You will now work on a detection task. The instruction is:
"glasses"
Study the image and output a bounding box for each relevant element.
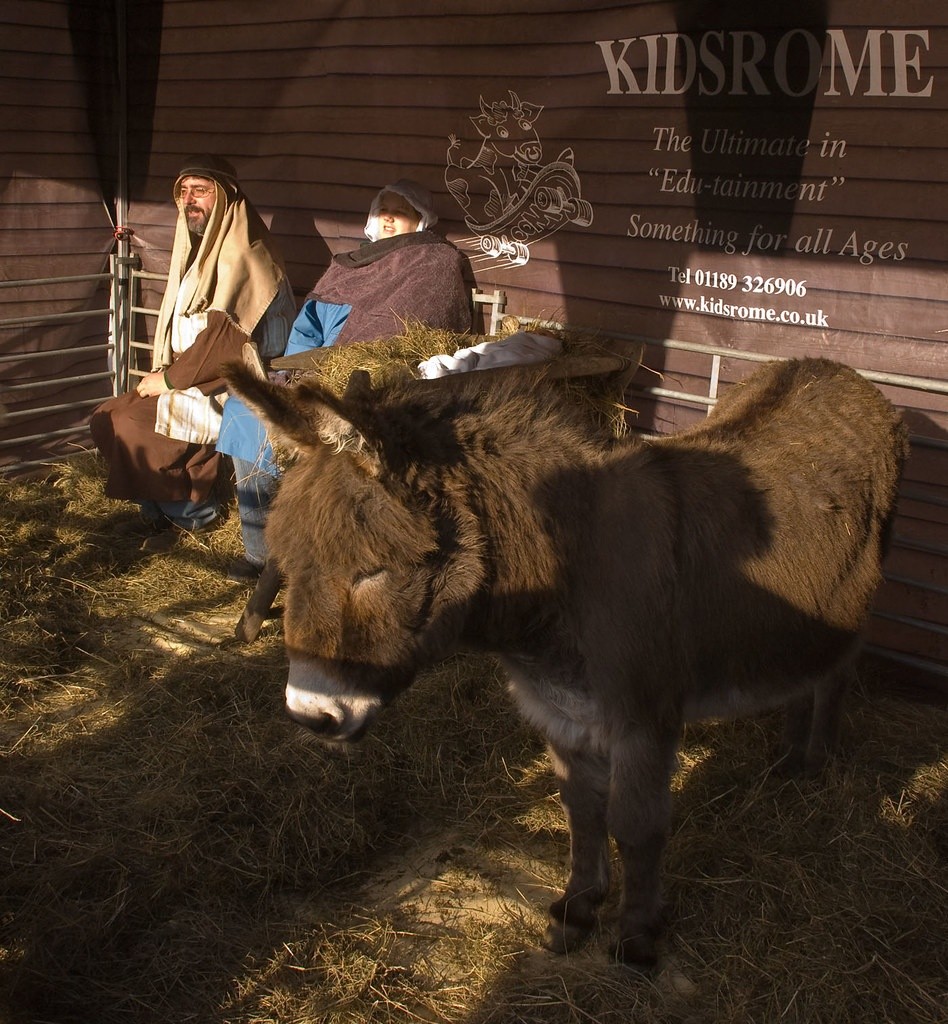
[177,187,215,198]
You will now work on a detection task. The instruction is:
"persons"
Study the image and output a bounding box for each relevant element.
[90,153,297,540]
[216,177,472,587]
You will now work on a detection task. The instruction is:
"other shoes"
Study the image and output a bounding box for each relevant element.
[143,513,219,553]
[226,558,261,578]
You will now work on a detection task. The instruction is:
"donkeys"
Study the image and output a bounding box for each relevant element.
[221,355,911,975]
